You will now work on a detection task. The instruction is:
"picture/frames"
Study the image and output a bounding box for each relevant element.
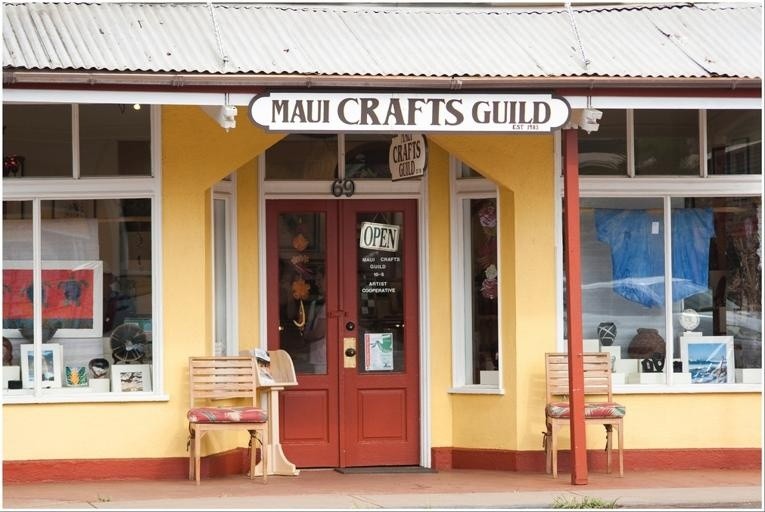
[680,335,736,384]
[2,259,152,392]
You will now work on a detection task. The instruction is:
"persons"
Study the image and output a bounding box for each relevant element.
[3,335,13,366]
[301,266,328,374]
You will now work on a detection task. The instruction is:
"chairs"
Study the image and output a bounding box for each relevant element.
[188,356,269,486]
[543,351,626,480]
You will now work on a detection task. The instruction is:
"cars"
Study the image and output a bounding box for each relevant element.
[563,277,762,370]
